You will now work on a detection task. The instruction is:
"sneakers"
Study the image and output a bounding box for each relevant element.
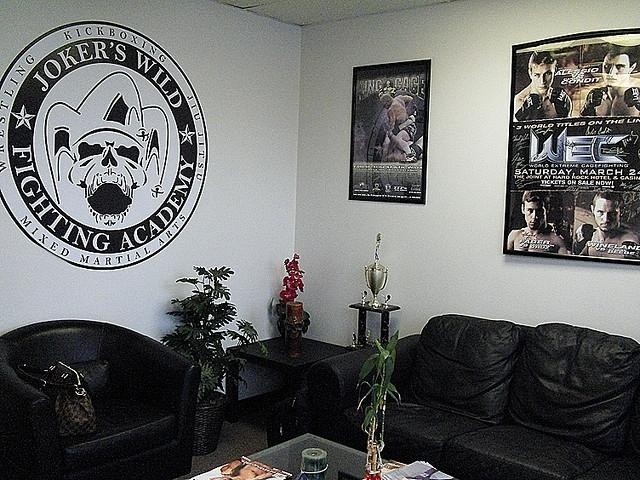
[399,156,417,163]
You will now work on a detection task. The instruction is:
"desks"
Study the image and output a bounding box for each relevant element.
[227,338,350,429]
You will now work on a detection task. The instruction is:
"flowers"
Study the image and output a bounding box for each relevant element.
[278,252,303,303]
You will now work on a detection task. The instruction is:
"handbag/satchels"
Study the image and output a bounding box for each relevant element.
[67,361,96,394]
[55,387,96,436]
[14,358,92,406]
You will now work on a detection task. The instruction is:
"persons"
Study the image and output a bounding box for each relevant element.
[514,51,573,121]
[212,460,274,480]
[379,93,417,164]
[580,50,640,117]
[575,192,639,258]
[508,190,571,254]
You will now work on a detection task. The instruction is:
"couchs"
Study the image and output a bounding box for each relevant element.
[306,314,639,480]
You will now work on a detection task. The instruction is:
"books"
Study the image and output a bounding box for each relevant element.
[381,458,454,480]
[189,455,293,480]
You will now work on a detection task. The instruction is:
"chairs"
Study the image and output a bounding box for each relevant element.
[1,318,200,479]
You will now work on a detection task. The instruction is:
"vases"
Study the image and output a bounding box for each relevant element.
[275,300,295,318]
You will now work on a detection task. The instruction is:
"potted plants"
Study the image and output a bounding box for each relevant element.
[159,264,272,457]
[355,331,399,480]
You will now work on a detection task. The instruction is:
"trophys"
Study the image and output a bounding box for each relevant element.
[363,232,389,309]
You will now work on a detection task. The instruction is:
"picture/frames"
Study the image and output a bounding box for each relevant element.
[503,27,640,266]
[349,58,431,205]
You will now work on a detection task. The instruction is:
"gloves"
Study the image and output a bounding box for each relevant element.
[622,241,640,259]
[550,87,571,117]
[574,223,596,254]
[516,94,542,121]
[553,220,572,251]
[579,88,602,116]
[624,87,640,111]
